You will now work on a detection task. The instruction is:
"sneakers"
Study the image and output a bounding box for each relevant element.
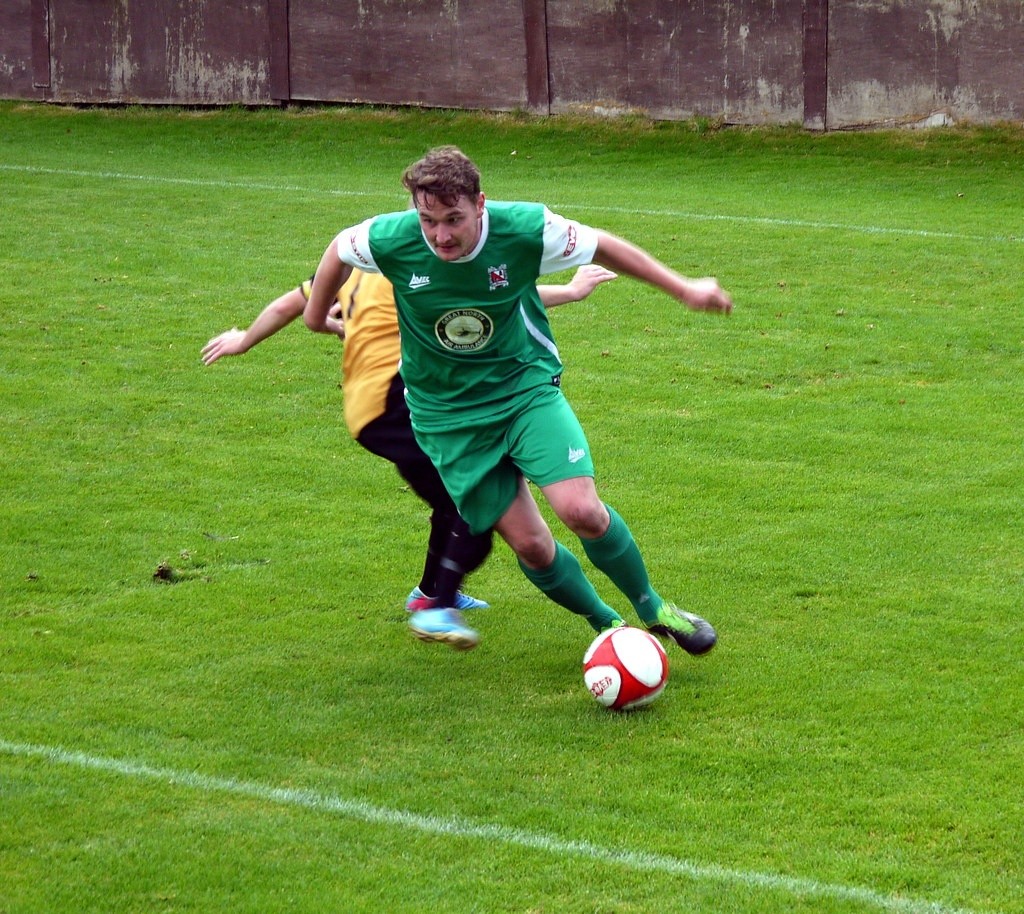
[404,585,491,615]
[640,598,719,657]
[597,620,629,636]
[406,606,481,649]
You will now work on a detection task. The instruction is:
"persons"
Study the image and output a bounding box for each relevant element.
[201,264,618,650]
[304,145,733,658]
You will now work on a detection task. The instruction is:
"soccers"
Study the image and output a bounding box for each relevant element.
[582,626,669,709]
